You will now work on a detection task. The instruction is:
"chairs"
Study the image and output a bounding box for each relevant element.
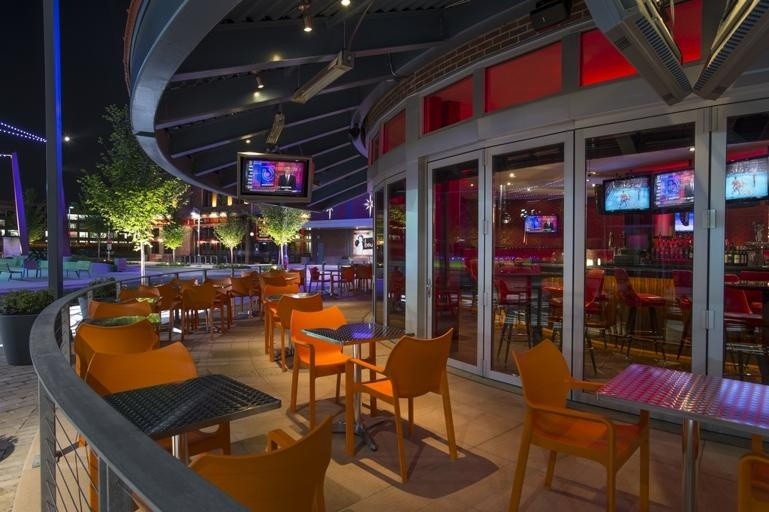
[508,337,649,512]
[738,435,769,512]
[263,284,301,355]
[290,307,376,429]
[85,287,161,326]
[131,414,333,512]
[74,319,161,448]
[155,263,373,341]
[83,343,231,512]
[345,327,457,482]
[389,242,769,375]
[274,295,323,370]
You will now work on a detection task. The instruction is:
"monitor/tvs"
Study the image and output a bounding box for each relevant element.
[653,167,695,215]
[725,154,769,204]
[237,151,315,204]
[602,174,653,215]
[523,214,559,234]
[673,211,694,234]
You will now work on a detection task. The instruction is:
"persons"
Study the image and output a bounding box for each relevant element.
[544,216,553,232]
[277,165,296,190]
[390,266,403,304]
[619,190,629,208]
[731,176,742,195]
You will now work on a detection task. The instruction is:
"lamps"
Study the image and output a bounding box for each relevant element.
[292,21,354,106]
[298,4,312,32]
[256,77,264,89]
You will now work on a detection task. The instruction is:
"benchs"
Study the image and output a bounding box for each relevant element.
[6,259,91,281]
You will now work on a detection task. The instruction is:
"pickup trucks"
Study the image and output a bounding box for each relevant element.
[493,265,544,305]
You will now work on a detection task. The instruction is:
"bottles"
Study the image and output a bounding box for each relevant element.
[651,236,769,271]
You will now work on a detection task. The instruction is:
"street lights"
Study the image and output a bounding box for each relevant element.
[68,207,73,252]
[190,206,202,267]
[283,207,289,272]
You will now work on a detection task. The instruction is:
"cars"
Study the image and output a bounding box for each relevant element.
[100,249,114,256]
[447,273,473,291]
[401,278,460,318]
[663,270,693,321]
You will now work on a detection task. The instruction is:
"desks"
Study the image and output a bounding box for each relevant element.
[300,324,415,451]
[102,373,282,466]
[598,361,769,512]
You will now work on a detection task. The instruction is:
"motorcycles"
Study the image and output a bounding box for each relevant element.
[388,283,405,314]
